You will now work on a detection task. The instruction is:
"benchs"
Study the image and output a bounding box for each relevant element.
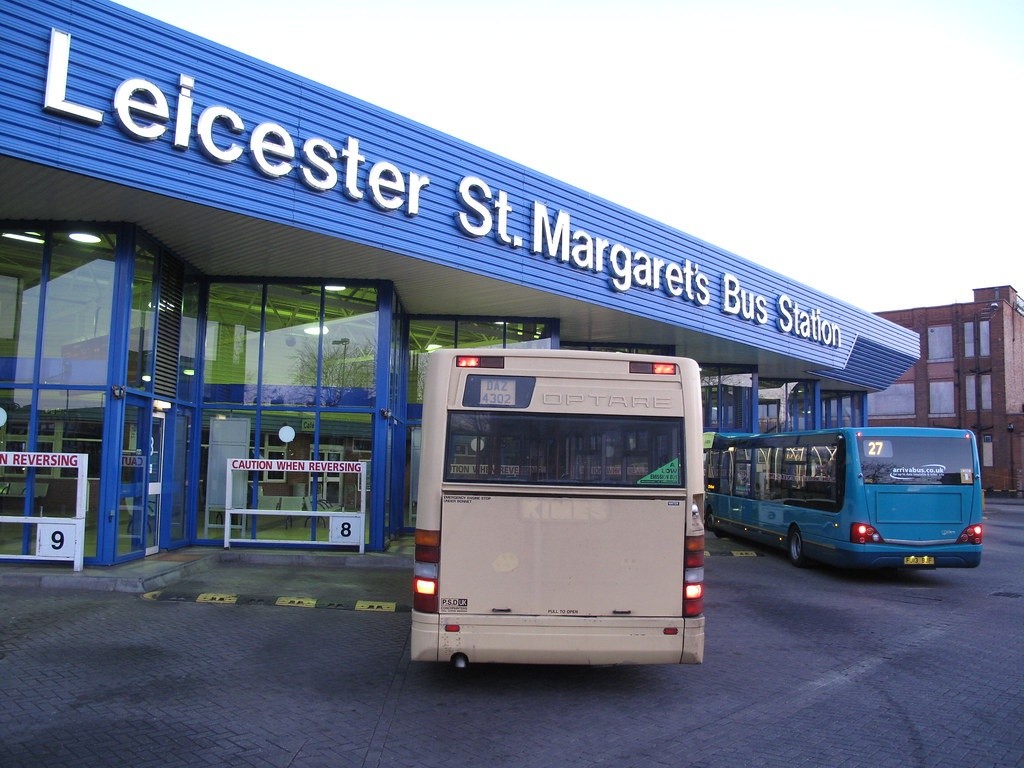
[0,482,49,517]
[257,496,304,530]
[304,495,341,528]
[124,497,156,534]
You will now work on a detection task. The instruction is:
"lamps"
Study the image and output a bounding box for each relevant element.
[110,383,127,400]
[380,405,393,419]
[279,416,296,443]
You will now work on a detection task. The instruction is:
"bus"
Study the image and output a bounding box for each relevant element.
[704,431,982,574]
[408,348,706,668]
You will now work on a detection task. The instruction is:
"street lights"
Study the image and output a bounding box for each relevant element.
[332,337,351,388]
